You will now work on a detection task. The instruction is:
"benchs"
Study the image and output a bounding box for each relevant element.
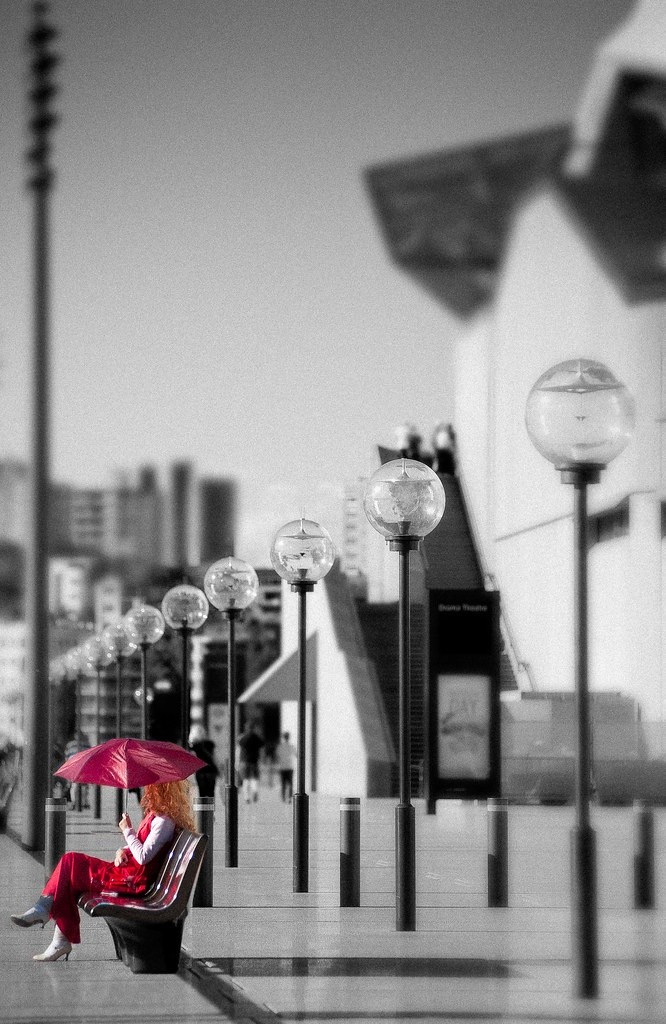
[75,832,211,973]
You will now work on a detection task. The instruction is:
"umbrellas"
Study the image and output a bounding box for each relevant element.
[52,737,211,817]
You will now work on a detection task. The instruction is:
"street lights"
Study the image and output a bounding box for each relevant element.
[203,551,260,868]
[361,458,446,933]
[159,582,211,753]
[97,621,139,829]
[62,646,89,812]
[49,658,65,800]
[121,602,166,739]
[266,518,340,895]
[82,634,117,819]
[524,356,640,998]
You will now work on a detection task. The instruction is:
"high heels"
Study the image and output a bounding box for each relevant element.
[32,924,73,961]
[10,895,54,930]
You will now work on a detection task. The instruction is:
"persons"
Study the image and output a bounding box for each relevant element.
[275,733,297,803]
[9,773,196,961]
[187,733,219,799]
[238,721,264,804]
